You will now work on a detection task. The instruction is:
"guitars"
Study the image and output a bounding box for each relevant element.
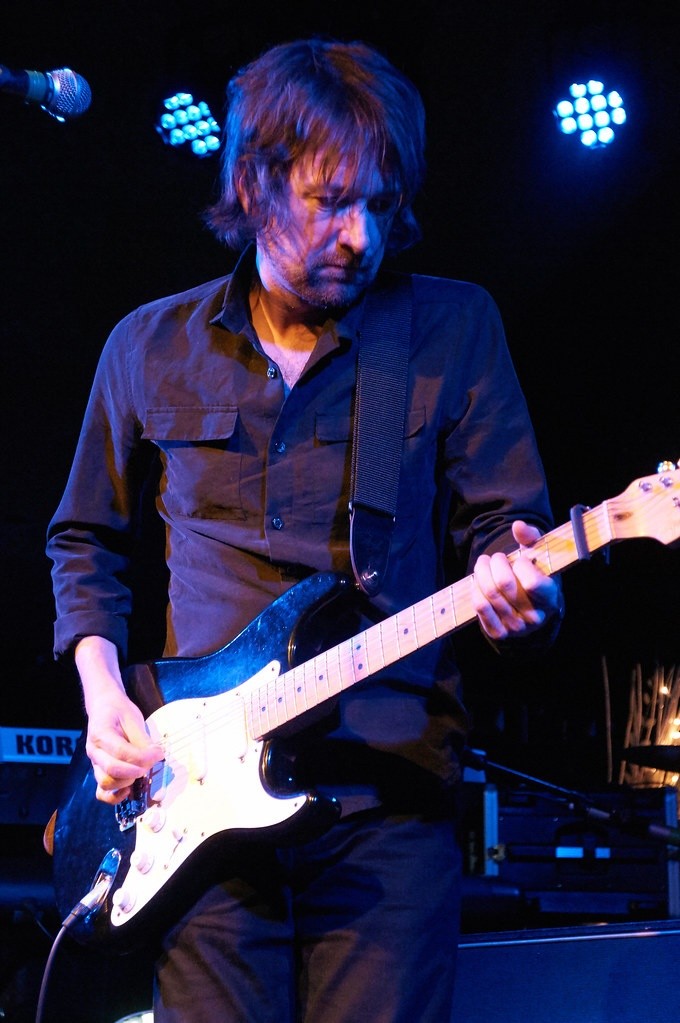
[50,454,679,956]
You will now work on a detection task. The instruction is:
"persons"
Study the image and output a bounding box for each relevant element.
[44,36,567,1023]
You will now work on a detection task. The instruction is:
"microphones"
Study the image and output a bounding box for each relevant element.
[0,65,92,121]
